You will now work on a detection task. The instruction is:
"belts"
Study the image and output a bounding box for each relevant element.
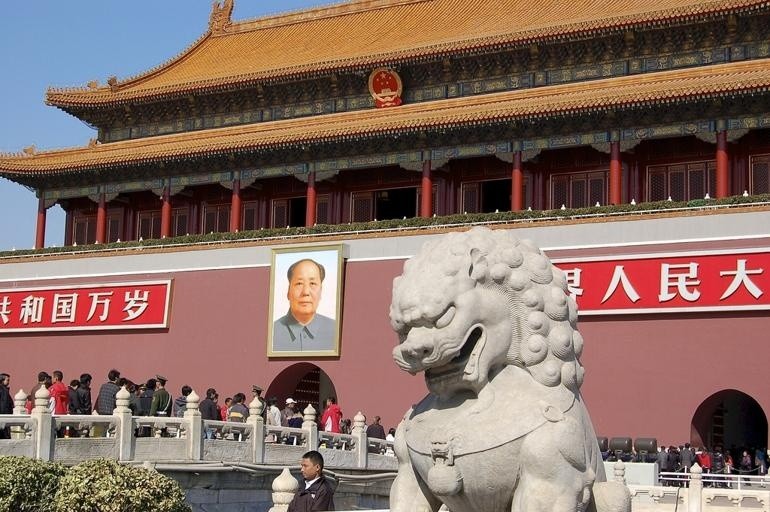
[156,411,168,414]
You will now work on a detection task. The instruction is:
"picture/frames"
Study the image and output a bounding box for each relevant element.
[265,243,344,361]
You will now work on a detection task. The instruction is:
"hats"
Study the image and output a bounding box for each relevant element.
[137,383,146,390]
[285,398,297,405]
[252,385,264,392]
[156,375,168,382]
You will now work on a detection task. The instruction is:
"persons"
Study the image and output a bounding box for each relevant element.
[601,443,770,489]
[287,451,335,512]
[273,260,336,351]
